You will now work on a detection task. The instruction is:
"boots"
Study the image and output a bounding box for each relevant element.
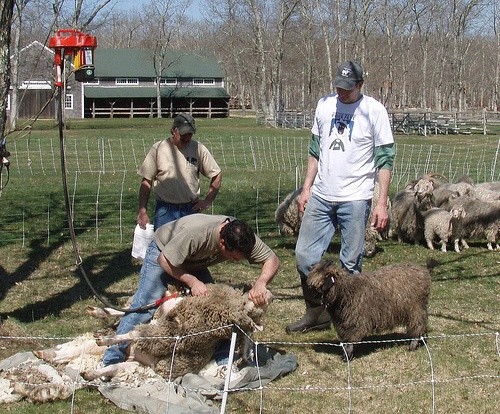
[286,277,331,335]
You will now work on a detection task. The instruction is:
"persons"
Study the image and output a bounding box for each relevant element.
[97,214,280,382]
[137,113,222,233]
[286,61,396,336]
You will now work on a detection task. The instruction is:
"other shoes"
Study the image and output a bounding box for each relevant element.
[218,363,239,379]
[97,362,112,382]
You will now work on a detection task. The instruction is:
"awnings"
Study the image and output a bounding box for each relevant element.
[84,87,231,99]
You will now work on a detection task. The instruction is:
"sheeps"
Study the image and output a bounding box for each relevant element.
[422,204,470,253]
[81,281,274,382]
[306,259,432,362]
[274,171,499,257]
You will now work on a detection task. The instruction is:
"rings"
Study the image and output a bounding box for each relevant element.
[200,208,201,210]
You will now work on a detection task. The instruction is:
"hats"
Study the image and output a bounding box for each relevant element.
[173,114,196,136]
[334,60,362,91]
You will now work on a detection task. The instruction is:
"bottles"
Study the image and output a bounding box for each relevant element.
[131,223,154,260]
